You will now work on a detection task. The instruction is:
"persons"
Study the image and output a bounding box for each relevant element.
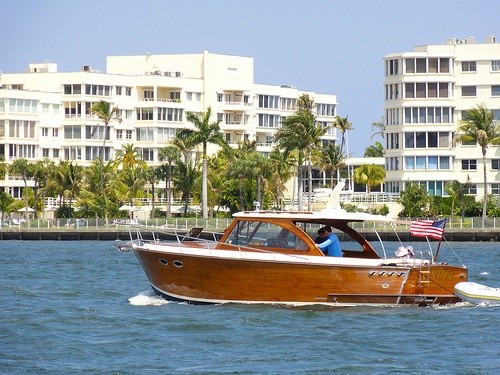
[315,228,328,256]
[312,225,344,257]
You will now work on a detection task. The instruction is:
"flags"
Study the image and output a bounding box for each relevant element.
[410,218,449,240]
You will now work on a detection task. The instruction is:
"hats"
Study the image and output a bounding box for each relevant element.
[394,246,408,257]
[406,245,415,256]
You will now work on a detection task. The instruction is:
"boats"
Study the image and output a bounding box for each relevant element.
[453,281,500,306]
[130,209,470,308]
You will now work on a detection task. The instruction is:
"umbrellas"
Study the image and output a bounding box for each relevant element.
[16,206,35,212]
